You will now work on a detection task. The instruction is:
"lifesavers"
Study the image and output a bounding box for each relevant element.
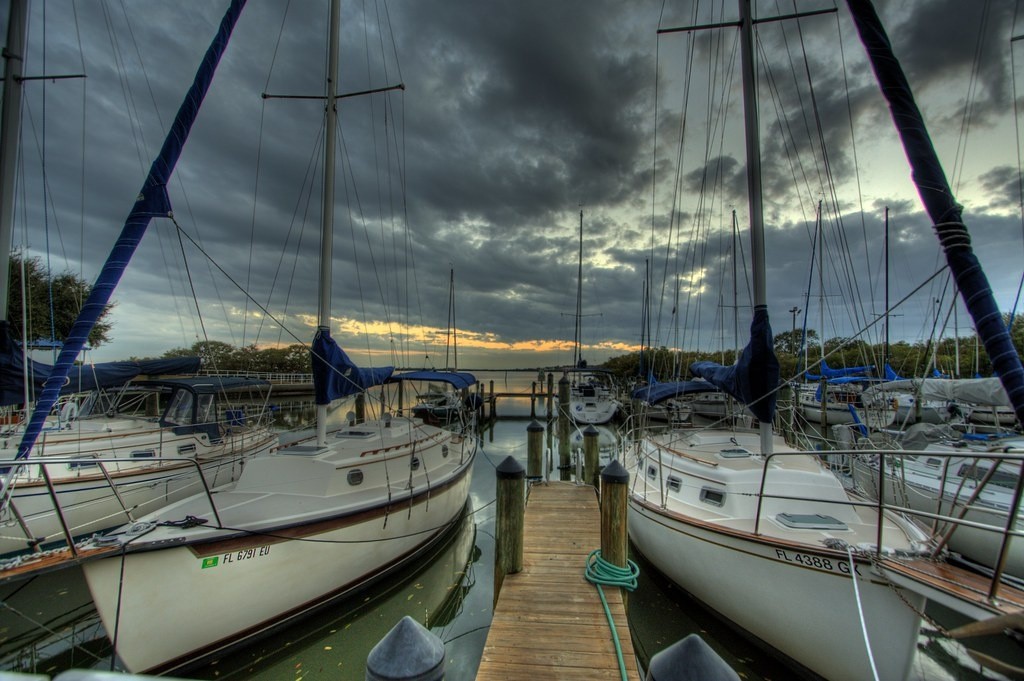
[59,401,77,422]
[892,398,898,408]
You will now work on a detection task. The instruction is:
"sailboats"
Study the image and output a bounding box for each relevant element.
[0,1,1024,681]
